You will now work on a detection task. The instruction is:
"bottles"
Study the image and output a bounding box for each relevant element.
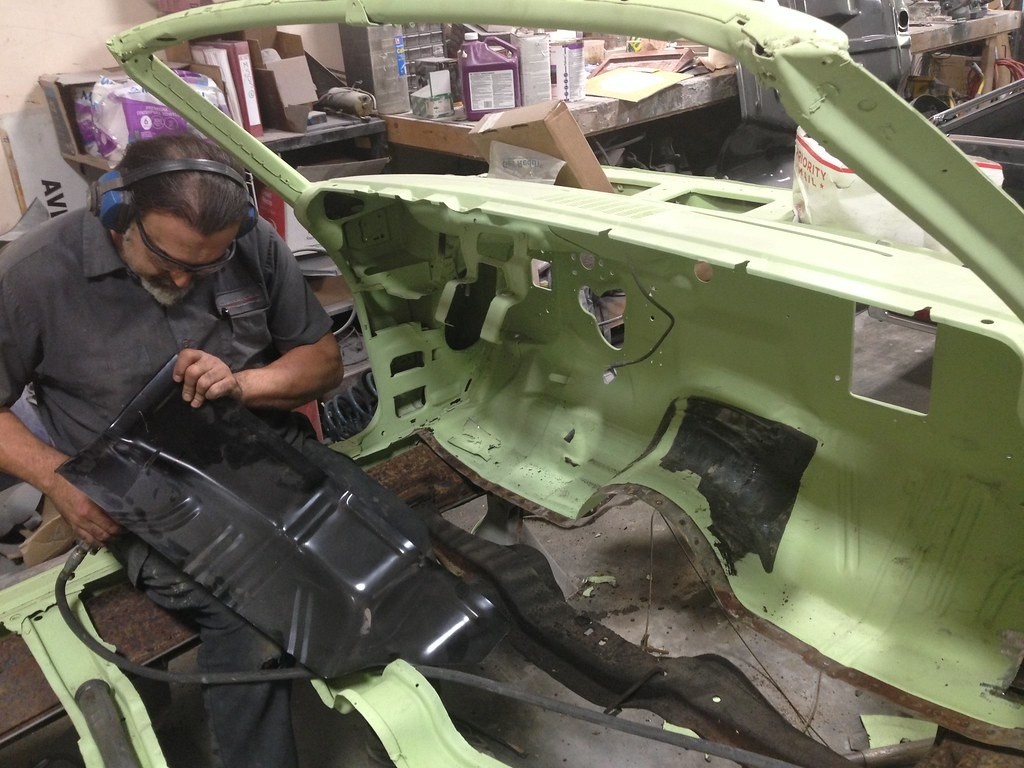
[457,32,522,122]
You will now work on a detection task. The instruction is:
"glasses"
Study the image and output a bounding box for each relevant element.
[132,214,238,278]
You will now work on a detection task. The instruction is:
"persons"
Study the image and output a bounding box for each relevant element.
[0,135,343,767]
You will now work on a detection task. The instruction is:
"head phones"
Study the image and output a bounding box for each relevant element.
[82,158,259,241]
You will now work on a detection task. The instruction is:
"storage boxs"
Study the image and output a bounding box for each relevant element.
[466,99,616,193]
[255,157,390,258]
[38,1,324,170]
